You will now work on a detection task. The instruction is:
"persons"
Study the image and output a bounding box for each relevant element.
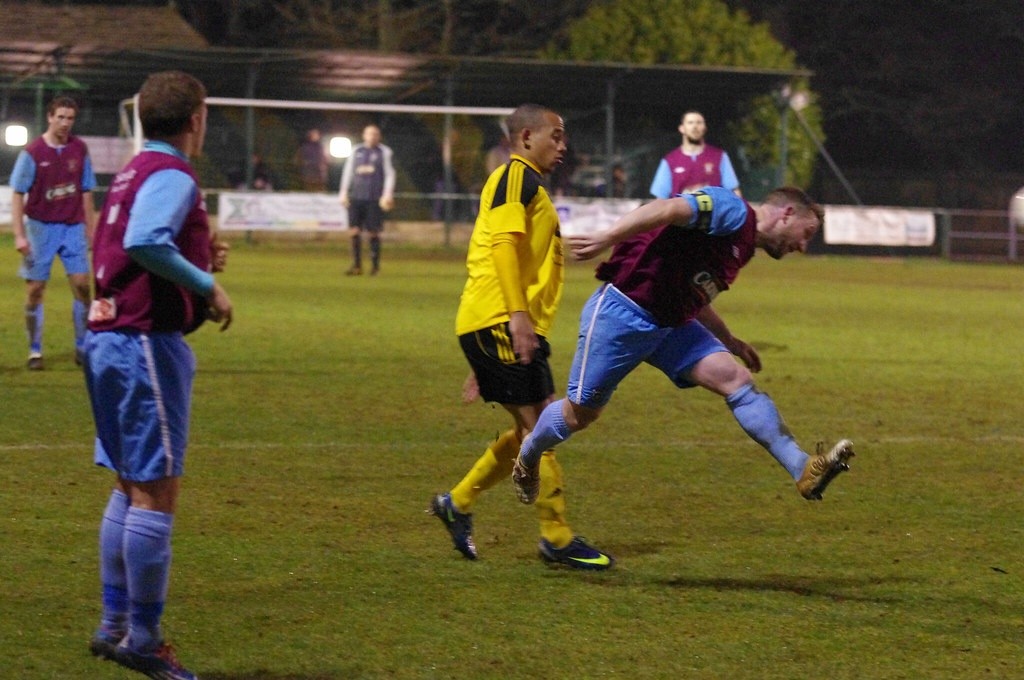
[431,105,615,572]
[10,96,98,371]
[339,124,395,277]
[650,110,742,200]
[513,187,857,505]
[238,128,643,220]
[82,71,233,680]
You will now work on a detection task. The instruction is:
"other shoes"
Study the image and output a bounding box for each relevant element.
[371,266,379,276]
[347,267,363,275]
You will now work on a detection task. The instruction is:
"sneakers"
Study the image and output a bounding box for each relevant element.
[27,353,42,370]
[113,634,198,680]
[536,535,616,570]
[75,350,84,364]
[796,439,856,501]
[512,432,540,505]
[89,619,128,661]
[425,491,480,560]
